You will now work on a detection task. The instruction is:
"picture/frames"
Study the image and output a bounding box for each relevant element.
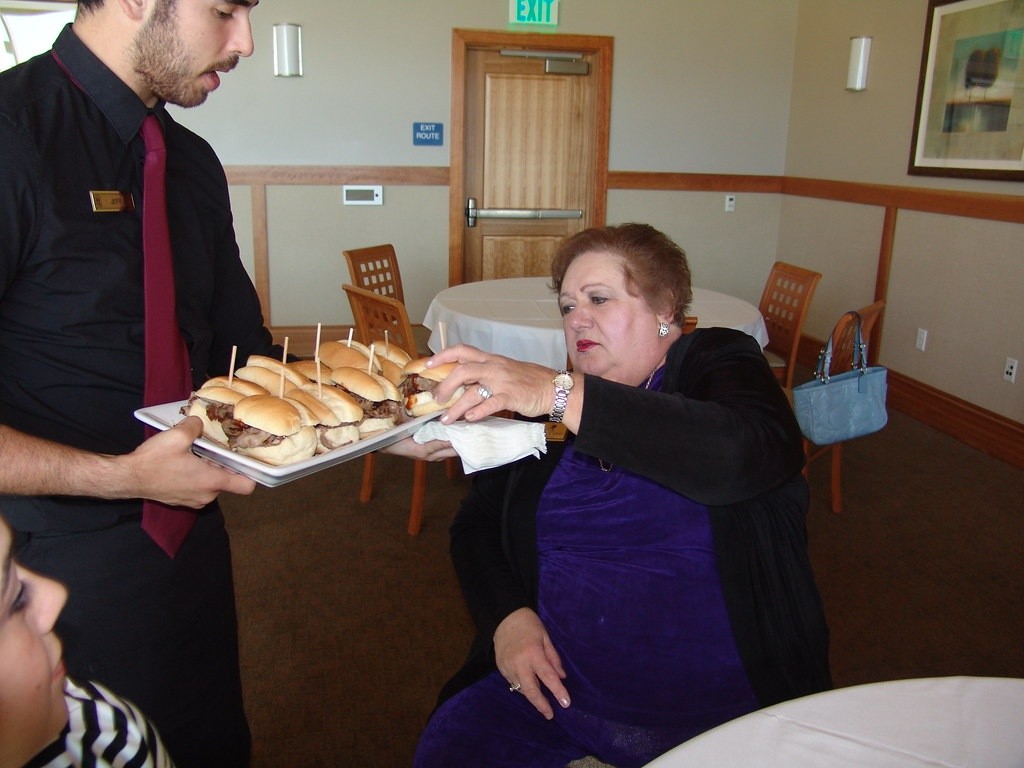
[906,0,1024,183]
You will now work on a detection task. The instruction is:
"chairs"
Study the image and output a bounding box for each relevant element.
[761,261,822,389]
[777,301,885,514]
[341,284,515,536]
[566,317,698,371]
[341,244,428,360]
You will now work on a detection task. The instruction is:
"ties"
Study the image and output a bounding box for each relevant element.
[53,54,201,559]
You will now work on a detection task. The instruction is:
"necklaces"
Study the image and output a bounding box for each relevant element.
[599,368,657,472]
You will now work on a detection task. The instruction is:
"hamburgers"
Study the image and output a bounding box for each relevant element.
[179,340,474,467]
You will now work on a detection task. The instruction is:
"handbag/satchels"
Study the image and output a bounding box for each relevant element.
[790,310,887,445]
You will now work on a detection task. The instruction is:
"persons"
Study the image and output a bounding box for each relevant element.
[0,0,461,768]
[410,223,831,768]
[0,516,176,768]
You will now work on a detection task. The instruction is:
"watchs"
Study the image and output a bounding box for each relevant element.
[549,370,574,423]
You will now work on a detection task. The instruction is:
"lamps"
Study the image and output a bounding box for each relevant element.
[271,23,304,77]
[844,35,872,92]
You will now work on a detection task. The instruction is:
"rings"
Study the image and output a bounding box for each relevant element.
[479,385,491,399]
[509,684,522,692]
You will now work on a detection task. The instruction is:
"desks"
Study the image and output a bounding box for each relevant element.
[423,277,769,374]
[640,675,1024,768]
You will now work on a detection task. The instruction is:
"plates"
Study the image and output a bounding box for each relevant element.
[134,400,450,488]
